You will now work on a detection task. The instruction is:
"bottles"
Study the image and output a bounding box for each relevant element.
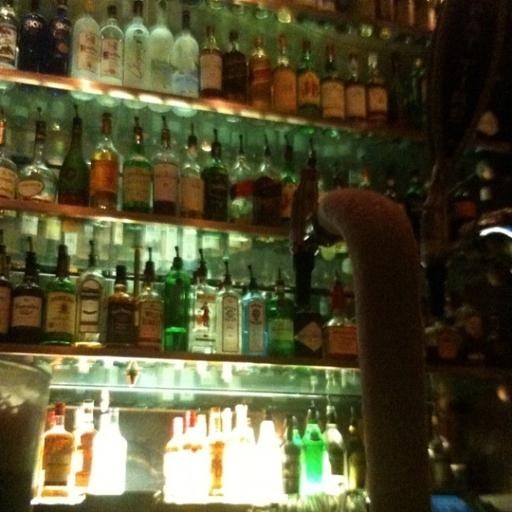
[366,56,390,132]
[187,269,220,354]
[202,141,230,220]
[73,1,100,82]
[468,333,509,368]
[42,244,76,347]
[255,145,281,226]
[0,246,12,342]
[323,423,347,509]
[162,257,189,351]
[359,172,372,191]
[407,176,430,240]
[478,187,495,218]
[216,273,242,355]
[249,35,271,111]
[135,261,164,351]
[60,116,89,207]
[273,32,296,115]
[75,255,105,347]
[0,0,18,71]
[43,413,54,434]
[455,301,483,342]
[0,121,17,200]
[346,52,367,127]
[268,286,294,358]
[162,403,300,508]
[452,182,476,229]
[223,30,249,103]
[241,279,270,357]
[124,1,149,87]
[106,265,136,347]
[46,0,71,76]
[296,38,322,120]
[280,145,302,225]
[72,405,97,496]
[38,403,76,498]
[123,126,152,213]
[425,321,462,363]
[323,287,360,363]
[385,177,399,204]
[90,112,120,212]
[20,121,57,203]
[230,154,253,225]
[181,135,204,219]
[100,4,122,85]
[151,1,173,95]
[306,150,316,168]
[293,313,326,358]
[173,10,199,100]
[22,0,44,73]
[151,128,180,216]
[89,407,128,496]
[10,252,45,344]
[200,25,222,97]
[426,411,470,494]
[322,45,346,124]
[302,419,325,496]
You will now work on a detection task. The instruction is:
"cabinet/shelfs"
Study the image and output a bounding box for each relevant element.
[1,0,512,512]
